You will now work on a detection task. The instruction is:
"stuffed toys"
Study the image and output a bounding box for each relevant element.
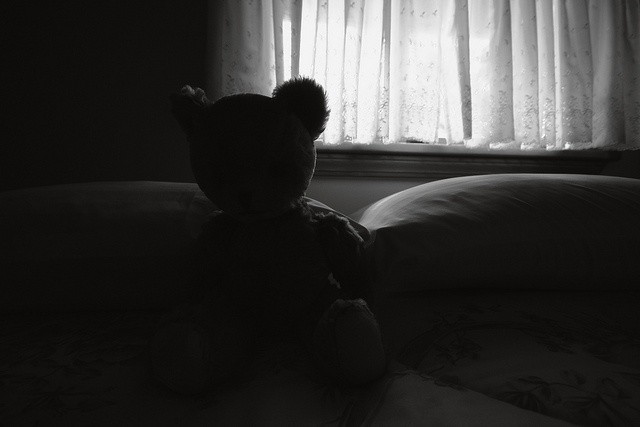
[148,79,388,399]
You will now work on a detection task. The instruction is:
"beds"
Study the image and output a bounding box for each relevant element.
[0,179,640,426]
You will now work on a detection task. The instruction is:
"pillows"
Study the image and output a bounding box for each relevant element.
[344,171,640,298]
[0,181,373,310]
